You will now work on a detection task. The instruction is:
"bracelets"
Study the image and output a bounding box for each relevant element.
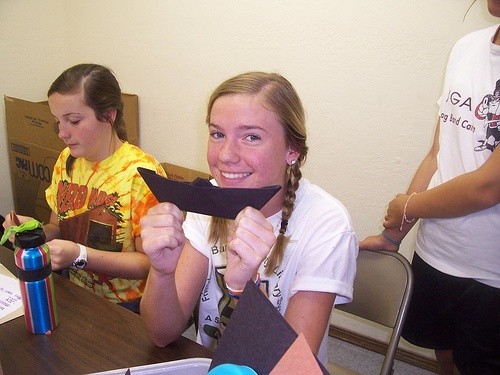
[400,192,417,231]
[226,272,261,299]
[381,231,401,245]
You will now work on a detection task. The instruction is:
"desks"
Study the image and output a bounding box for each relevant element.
[0,245,216,375]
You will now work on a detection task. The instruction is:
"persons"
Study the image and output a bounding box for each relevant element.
[356,0,500,375]
[2,63,170,316]
[140,71,359,366]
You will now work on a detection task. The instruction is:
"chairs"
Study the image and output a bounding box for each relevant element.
[327,249,414,375]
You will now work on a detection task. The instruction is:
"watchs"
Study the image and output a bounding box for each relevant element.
[72,243,88,270]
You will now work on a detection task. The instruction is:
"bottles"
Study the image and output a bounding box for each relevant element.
[13,227,59,335]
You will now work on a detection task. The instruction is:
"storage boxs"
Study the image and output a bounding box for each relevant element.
[3,93,141,225]
